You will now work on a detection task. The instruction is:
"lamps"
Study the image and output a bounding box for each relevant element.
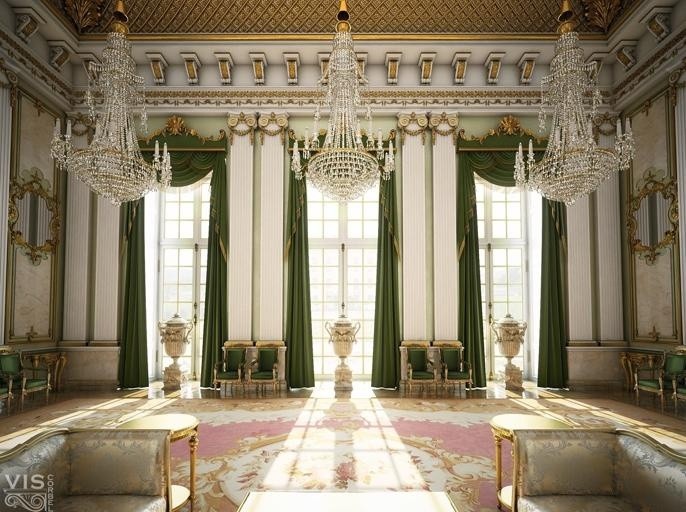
[48,0,174,208]
[290,0,396,206]
[512,0,636,208]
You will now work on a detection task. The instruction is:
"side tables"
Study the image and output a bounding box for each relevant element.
[24,351,69,393]
[488,413,571,512]
[619,351,662,393]
[116,414,200,512]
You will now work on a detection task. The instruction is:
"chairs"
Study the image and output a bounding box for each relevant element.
[0,344,50,413]
[672,372,686,414]
[247,348,279,396]
[213,348,247,393]
[0,374,18,417]
[438,347,473,395]
[632,345,686,413]
[406,348,435,393]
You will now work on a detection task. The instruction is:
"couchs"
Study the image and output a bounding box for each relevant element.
[0,428,172,512]
[511,427,686,512]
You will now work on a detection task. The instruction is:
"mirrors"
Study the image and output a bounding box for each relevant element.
[625,166,678,265]
[8,167,63,266]
